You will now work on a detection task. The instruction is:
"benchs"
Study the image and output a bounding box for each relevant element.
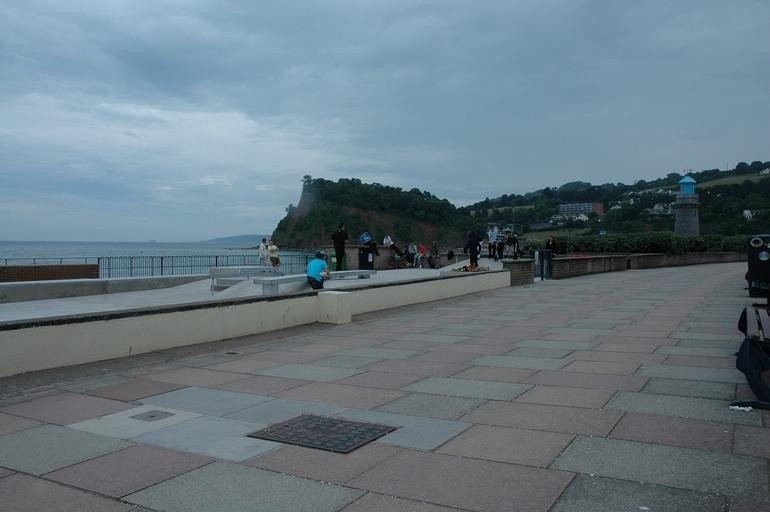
[253,270,376,297]
[389,257,440,268]
[211,267,283,297]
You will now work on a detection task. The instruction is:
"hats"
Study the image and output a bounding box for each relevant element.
[316,251,325,258]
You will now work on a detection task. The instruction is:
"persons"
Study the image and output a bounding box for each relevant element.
[545,234,556,256]
[258,238,271,273]
[268,240,281,268]
[359,229,381,256]
[329,221,349,271]
[406,240,439,268]
[304,250,331,290]
[463,225,526,268]
[383,233,404,257]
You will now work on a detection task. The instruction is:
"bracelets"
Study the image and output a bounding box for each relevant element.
[326,272,329,274]
